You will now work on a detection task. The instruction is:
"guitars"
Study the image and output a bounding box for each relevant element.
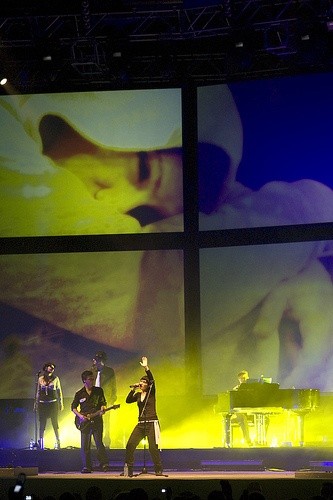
[75,404,120,430]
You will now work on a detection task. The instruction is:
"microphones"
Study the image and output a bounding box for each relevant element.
[130,384,142,387]
[35,370,41,376]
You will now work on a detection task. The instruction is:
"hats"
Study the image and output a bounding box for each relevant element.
[43,363,55,371]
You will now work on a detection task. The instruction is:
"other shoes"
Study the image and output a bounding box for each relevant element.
[155,470,162,475]
[121,469,133,476]
[247,440,253,446]
[101,464,109,471]
[54,442,60,451]
[81,466,90,473]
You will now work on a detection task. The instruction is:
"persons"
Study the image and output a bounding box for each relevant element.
[119,355,163,476]
[233,370,269,446]
[71,370,109,474]
[91,351,117,447]
[0,85,333,400]
[32,362,64,450]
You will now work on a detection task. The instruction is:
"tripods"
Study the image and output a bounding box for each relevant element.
[130,388,168,477]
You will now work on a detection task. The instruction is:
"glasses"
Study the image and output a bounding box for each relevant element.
[140,381,147,383]
[87,378,95,381]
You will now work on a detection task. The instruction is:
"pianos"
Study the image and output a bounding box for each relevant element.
[213,377,321,448]
